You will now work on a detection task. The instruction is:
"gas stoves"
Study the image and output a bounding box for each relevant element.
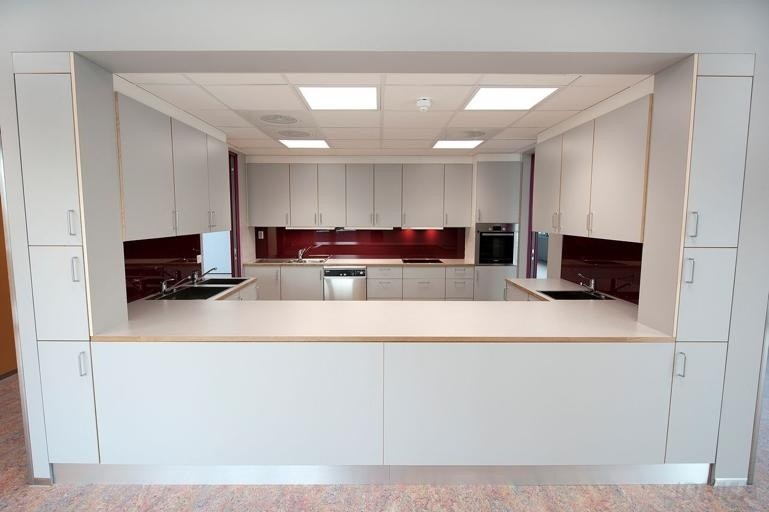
[401,256,445,265]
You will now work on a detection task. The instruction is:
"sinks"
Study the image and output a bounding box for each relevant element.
[200,278,246,284]
[540,290,615,300]
[255,259,287,263]
[286,258,324,264]
[160,287,230,300]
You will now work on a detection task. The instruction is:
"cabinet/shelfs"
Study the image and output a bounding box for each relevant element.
[678,251,737,342]
[683,76,753,248]
[28,246,90,341]
[14,72,81,246]
[36,340,100,464]
[664,340,728,466]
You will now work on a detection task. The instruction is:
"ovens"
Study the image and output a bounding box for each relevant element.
[476,223,519,266]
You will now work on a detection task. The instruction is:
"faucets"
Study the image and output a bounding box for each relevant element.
[578,272,599,292]
[298,247,310,260]
[159,275,191,294]
[192,267,217,283]
[610,272,636,293]
